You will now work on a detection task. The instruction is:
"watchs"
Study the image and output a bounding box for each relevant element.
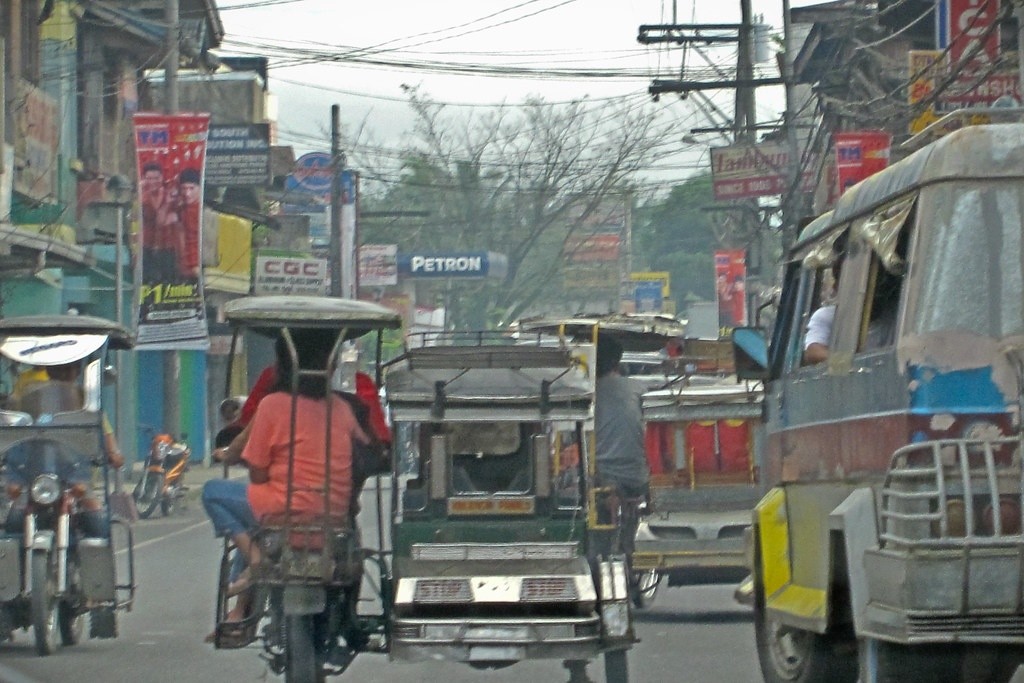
[222,447,229,457]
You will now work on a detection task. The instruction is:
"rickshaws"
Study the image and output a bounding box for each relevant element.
[211,295,642,683]
[582,337,764,608]
[0,309,139,657]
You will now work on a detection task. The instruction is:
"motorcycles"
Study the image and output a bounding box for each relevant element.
[730,117,1023,683]
[131,429,192,518]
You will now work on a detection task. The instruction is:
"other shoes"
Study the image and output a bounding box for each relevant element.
[205,612,251,642]
[226,556,276,598]
[339,617,372,649]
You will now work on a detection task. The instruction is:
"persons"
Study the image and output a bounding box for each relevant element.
[203,327,391,645]
[799,246,901,363]
[558,332,647,575]
[133,229,143,231]
[140,162,199,306]
[11,358,125,482]
[719,268,746,327]
